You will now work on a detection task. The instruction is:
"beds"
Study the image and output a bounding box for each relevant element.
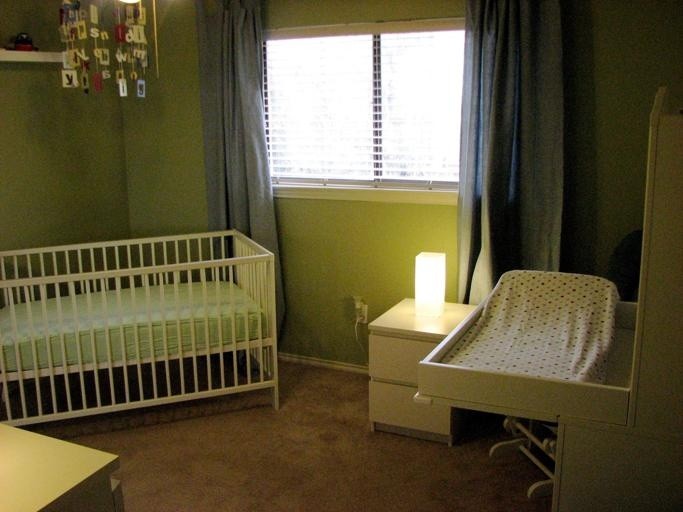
[0,229,280,426]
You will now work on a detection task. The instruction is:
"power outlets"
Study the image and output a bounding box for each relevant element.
[355,305,368,325]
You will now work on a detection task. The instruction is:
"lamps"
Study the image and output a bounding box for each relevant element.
[415,252,446,317]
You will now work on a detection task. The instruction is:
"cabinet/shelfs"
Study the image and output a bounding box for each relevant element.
[413,82,683,512]
[367,298,481,447]
[0,422,125,512]
